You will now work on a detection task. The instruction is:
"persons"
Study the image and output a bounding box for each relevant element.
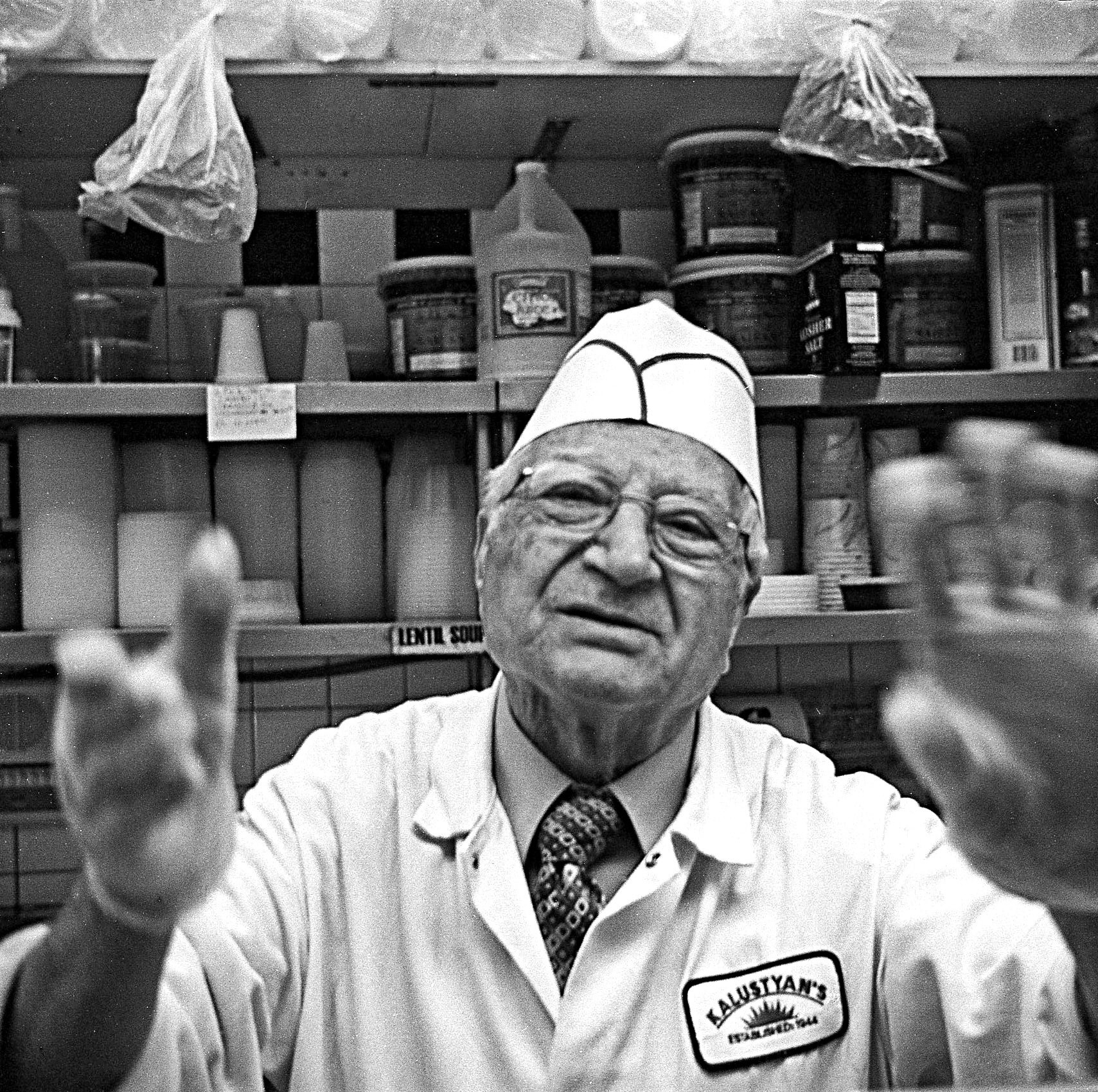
[0,297,1097,1092]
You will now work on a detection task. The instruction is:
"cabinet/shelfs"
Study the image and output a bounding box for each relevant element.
[0,367,1098,665]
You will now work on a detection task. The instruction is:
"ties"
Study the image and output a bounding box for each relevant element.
[529,790,623,995]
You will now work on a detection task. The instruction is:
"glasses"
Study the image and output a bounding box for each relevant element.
[495,459,754,579]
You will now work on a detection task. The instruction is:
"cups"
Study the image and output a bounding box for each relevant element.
[759,414,921,584]
[16,418,477,630]
[214,306,269,385]
[302,320,349,382]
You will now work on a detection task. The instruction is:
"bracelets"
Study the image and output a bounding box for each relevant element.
[84,857,180,938]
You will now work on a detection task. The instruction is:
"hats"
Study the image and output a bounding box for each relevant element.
[511,299,762,510]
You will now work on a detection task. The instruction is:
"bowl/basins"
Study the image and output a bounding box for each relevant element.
[179,294,266,380]
[66,260,161,384]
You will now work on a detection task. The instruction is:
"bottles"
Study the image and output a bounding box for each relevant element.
[475,160,592,379]
[0,184,78,384]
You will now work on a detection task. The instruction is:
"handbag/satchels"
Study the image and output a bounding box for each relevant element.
[772,14,947,168]
[75,8,258,248]
